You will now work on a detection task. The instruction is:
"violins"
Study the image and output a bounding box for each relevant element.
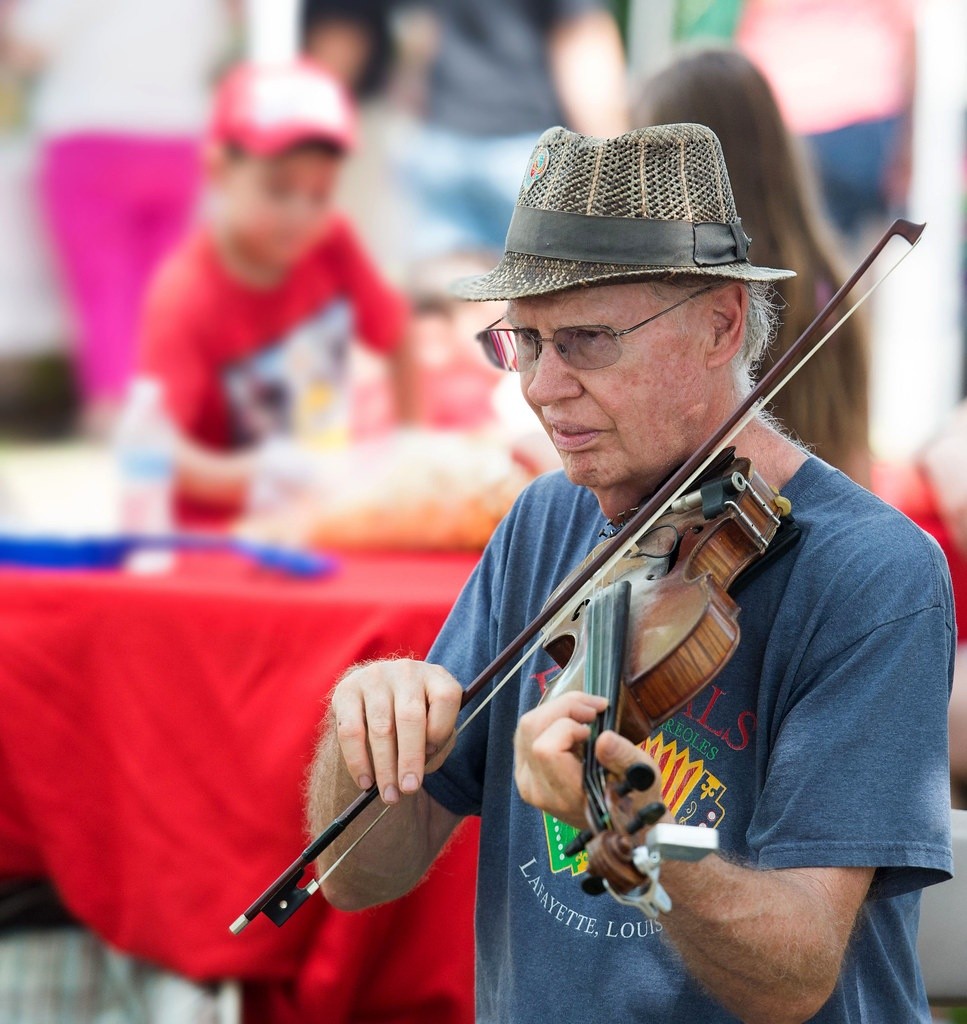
[540,454,800,925]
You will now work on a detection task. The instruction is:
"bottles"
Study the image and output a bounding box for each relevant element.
[115,378,174,571]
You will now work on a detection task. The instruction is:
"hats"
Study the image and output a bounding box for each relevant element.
[214,61,357,158]
[457,122,796,304]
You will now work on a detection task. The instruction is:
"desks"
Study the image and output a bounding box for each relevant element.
[0,509,967,1024]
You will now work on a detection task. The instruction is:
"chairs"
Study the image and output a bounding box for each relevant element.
[37,125,210,446]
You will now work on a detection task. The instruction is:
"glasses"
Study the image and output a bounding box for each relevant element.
[474,280,720,374]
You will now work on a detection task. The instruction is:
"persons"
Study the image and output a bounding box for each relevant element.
[130,60,418,535]
[306,123,956,1024]
[631,49,873,481]
[310,1,627,473]
